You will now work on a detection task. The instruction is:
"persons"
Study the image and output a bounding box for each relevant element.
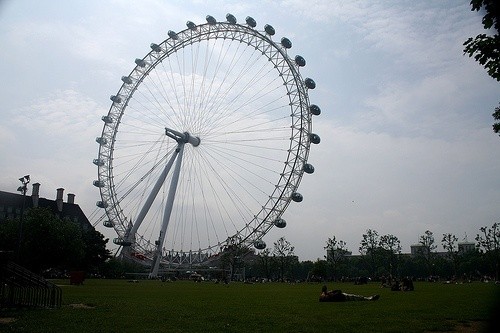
[159,268,499,290]
[319,286,380,302]
